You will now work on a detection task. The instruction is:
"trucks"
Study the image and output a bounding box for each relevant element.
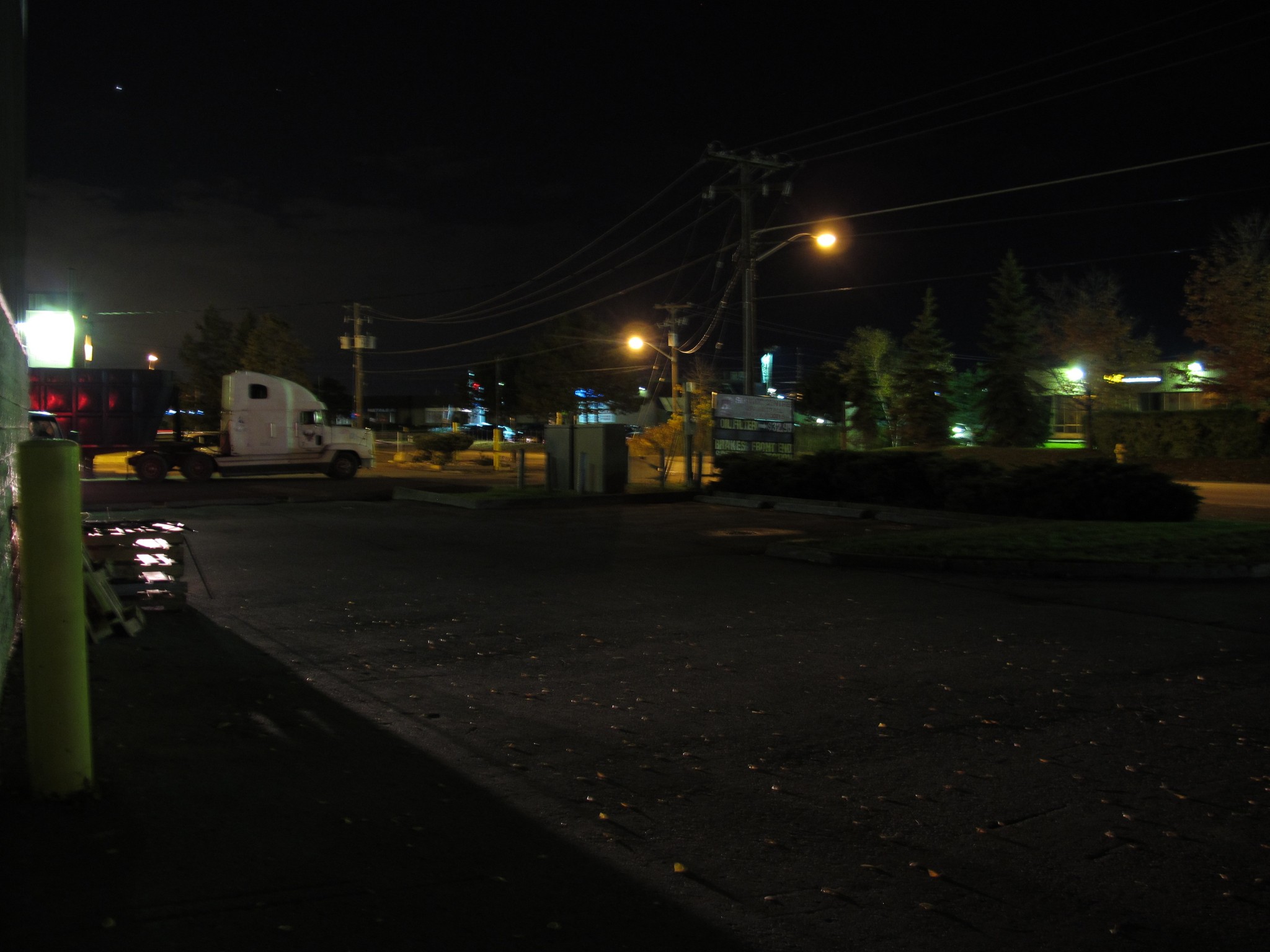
[25,369,376,484]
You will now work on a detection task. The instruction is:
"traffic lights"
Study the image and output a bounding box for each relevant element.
[494,358,518,409]
[467,355,487,398]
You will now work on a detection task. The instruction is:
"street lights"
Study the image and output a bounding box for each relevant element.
[627,336,677,435]
[1068,368,1092,448]
[742,233,838,396]
